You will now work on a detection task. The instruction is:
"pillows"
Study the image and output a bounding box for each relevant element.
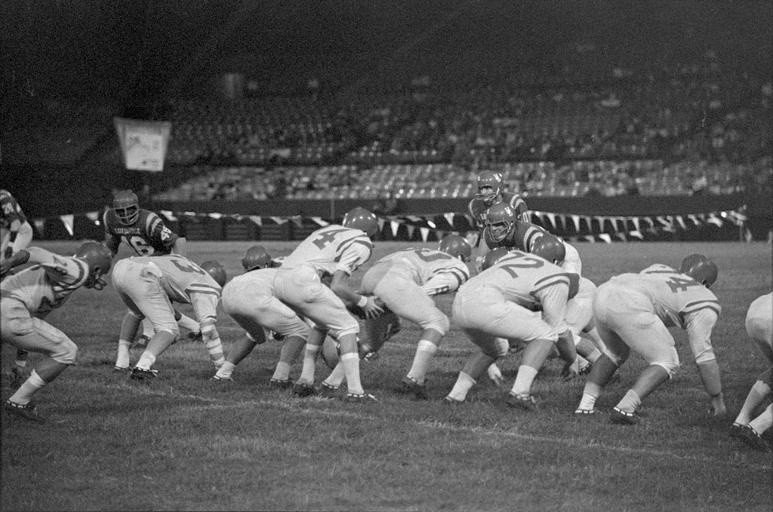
[75,241,113,290]
[200,260,226,287]
[484,203,518,243]
[343,207,378,237]
[531,236,565,266]
[242,246,272,272]
[112,191,139,226]
[437,235,472,265]
[472,171,504,204]
[679,254,718,289]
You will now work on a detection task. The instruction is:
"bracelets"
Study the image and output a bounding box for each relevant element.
[357,295,368,306]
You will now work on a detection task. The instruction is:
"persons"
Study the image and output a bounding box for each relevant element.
[242,245,340,369]
[101,191,201,347]
[5,78,773,206]
[728,290,773,444]
[273,208,382,404]
[361,237,474,400]
[212,268,310,389]
[7,181,8,184]
[444,236,578,412]
[468,168,530,272]
[574,254,726,424]
[0,189,36,267]
[479,203,582,275]
[0,240,111,421]
[113,255,228,381]
[486,248,609,376]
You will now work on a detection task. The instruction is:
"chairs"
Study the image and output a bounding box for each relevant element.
[10,362,25,390]
[3,399,46,425]
[400,371,436,402]
[268,378,296,392]
[101,89,772,200]
[728,422,772,453]
[572,405,646,426]
[112,365,160,385]
[441,389,542,415]
[293,379,380,399]
[209,374,237,384]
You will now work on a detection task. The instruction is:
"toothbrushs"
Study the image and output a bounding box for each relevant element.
[347,294,386,318]
